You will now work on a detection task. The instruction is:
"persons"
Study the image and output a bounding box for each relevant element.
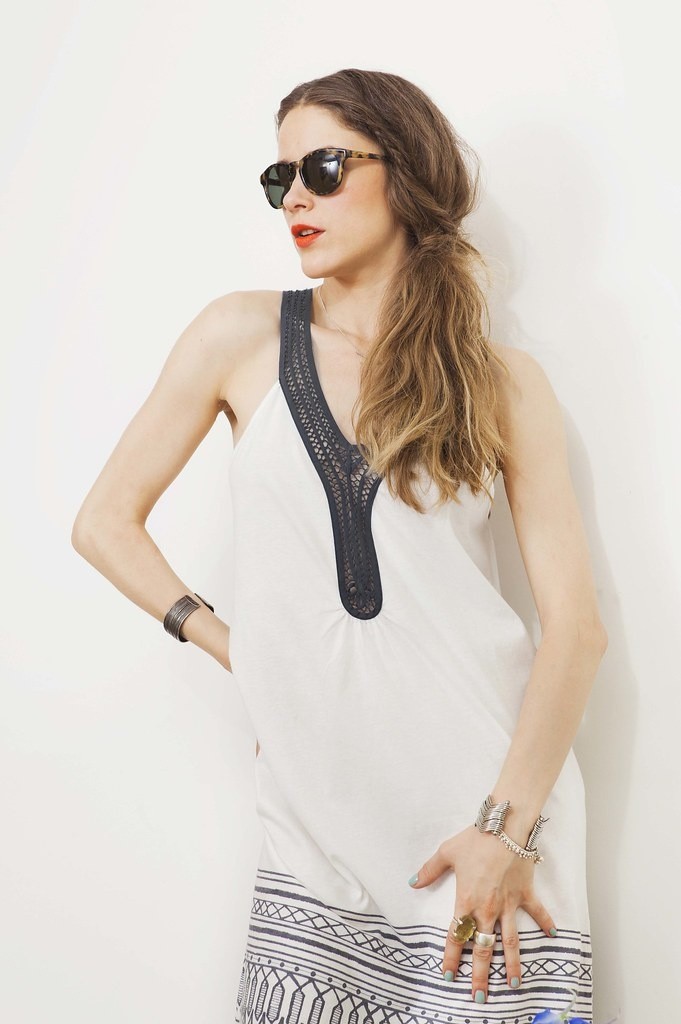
[68,69,610,1024]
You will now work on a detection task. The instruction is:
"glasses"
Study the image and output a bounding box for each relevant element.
[261,148,393,209]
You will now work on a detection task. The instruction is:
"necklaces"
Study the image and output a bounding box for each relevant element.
[317,285,372,359]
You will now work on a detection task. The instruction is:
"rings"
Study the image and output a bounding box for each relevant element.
[473,930,497,948]
[451,914,477,942]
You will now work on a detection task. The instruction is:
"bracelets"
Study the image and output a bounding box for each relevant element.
[474,794,550,865]
[164,592,215,643]
